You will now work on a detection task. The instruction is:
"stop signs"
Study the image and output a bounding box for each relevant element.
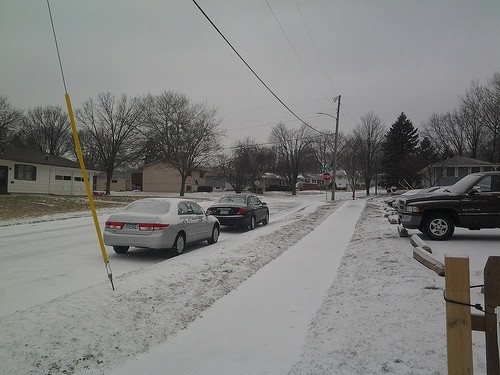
[322,172,331,181]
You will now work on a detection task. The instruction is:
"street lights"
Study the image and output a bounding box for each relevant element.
[315,95,341,201]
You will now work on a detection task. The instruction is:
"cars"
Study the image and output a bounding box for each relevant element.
[103,197,221,257]
[206,192,269,231]
[397,170,500,242]
